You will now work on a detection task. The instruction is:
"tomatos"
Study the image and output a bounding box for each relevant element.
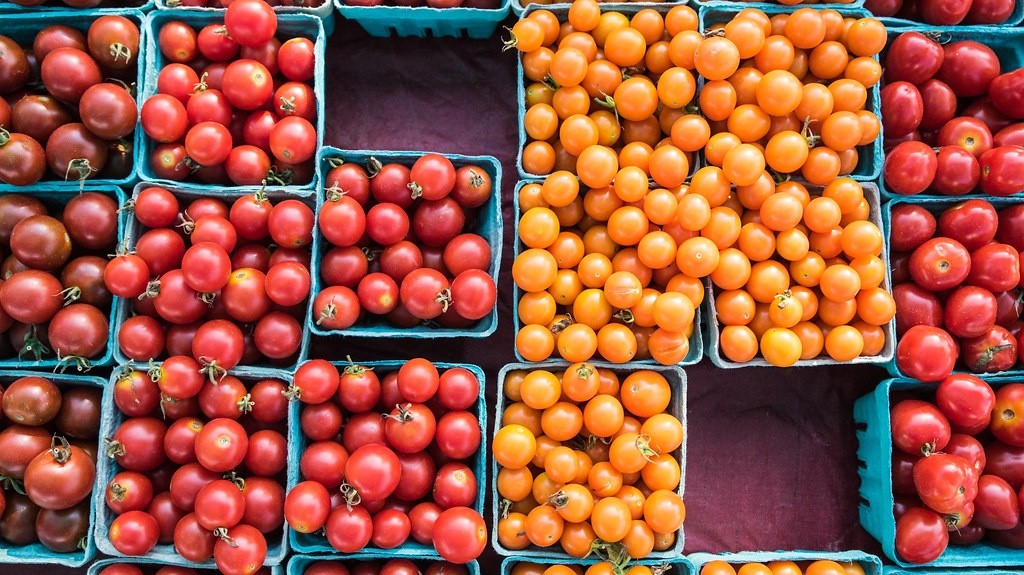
[492,0,1024,575]
[0,0,497,575]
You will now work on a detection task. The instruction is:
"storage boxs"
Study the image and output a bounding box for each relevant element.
[0,5,1024,573]
